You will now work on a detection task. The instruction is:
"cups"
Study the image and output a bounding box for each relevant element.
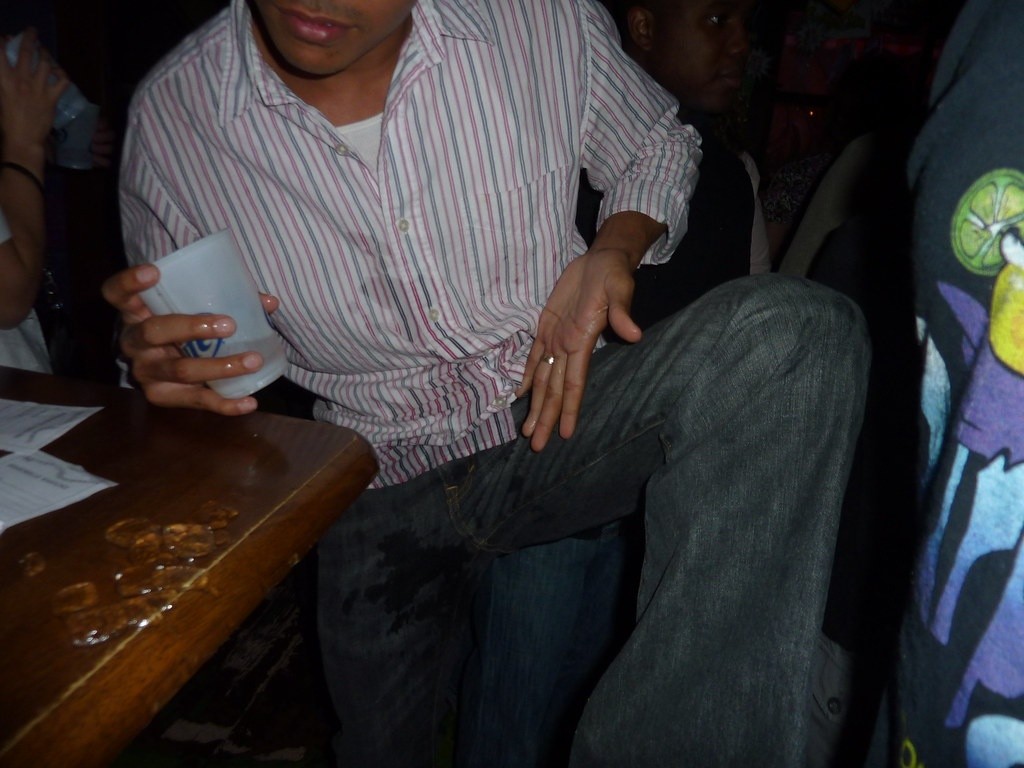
[133,227,287,400]
[61,103,100,169]
[5,31,87,130]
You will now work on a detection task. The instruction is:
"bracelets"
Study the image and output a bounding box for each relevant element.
[0,161,44,193]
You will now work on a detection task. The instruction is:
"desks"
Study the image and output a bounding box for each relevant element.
[0,365,380,768]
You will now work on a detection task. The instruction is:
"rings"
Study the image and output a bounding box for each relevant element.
[541,356,555,364]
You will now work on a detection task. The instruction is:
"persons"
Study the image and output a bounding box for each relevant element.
[0,27,115,401]
[104,1,871,768]
[869,2,1024,767]
[576,0,773,345]
